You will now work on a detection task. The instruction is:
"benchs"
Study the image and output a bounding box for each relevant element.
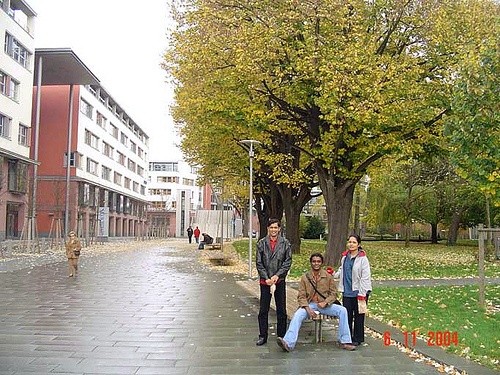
[237,281,334,345]
[204,250,227,265]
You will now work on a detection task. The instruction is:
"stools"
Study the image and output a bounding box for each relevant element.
[208,243,220,249]
[216,237,230,243]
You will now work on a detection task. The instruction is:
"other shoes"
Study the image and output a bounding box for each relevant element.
[277,337,290,352]
[340,343,357,351]
[256,337,267,346]
[361,342,368,347]
[69,274,73,277]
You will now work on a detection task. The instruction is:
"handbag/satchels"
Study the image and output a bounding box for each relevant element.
[334,300,341,306]
[74,251,80,256]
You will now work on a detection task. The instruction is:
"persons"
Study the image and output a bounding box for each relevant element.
[276,254,356,352]
[187,226,213,250]
[65,232,81,276]
[256,219,292,347]
[325,235,372,347]
